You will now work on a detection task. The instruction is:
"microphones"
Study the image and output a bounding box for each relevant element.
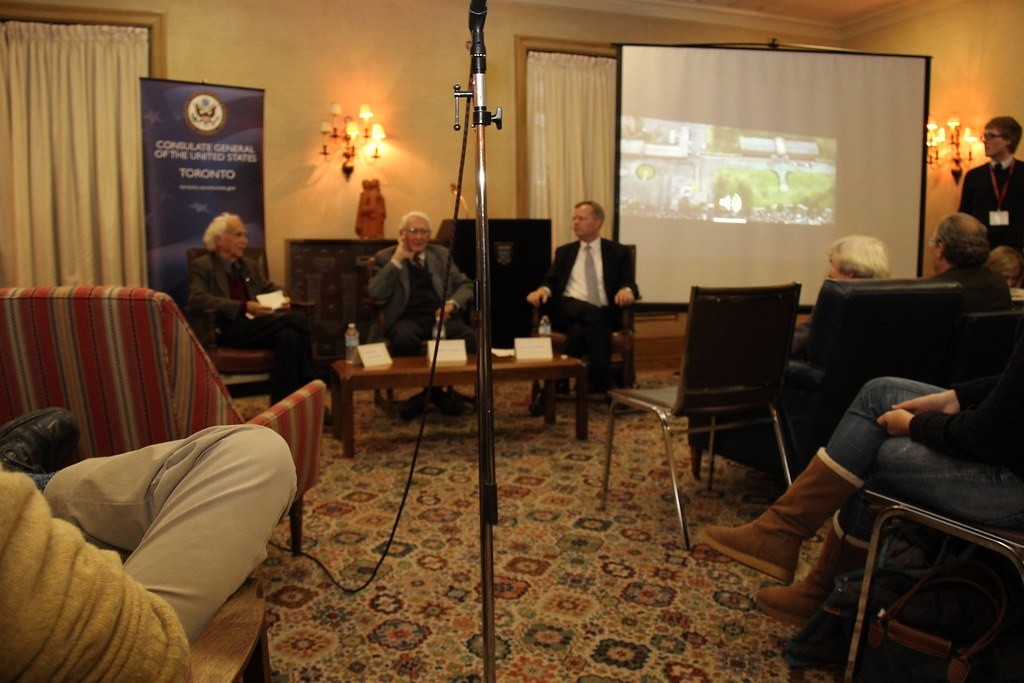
[468,0,486,34]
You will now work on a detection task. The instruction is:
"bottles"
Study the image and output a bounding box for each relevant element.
[345,324,360,366]
[538,315,550,338]
[433,317,446,340]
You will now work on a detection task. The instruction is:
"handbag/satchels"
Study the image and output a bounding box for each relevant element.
[785,557,1007,683]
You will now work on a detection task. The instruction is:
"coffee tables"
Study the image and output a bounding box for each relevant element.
[333,351,588,457]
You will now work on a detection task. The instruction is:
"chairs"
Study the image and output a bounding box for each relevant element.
[0,243,1024,683]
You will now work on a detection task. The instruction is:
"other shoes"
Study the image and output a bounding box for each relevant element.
[402,392,429,419]
[439,389,463,414]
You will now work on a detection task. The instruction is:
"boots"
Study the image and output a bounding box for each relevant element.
[755,508,871,626]
[699,446,865,583]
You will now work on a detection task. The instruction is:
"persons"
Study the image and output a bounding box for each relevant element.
[0,408,297,683]
[928,213,1014,313]
[959,116,1024,288]
[700,313,1024,626]
[367,211,476,421]
[791,235,891,353]
[527,201,639,416]
[984,247,1024,288]
[187,212,332,425]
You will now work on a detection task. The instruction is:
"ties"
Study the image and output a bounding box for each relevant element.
[585,245,601,307]
[413,256,422,268]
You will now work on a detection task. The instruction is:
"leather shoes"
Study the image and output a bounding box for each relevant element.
[0,407,81,473]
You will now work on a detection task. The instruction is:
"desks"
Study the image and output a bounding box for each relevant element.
[284,237,435,392]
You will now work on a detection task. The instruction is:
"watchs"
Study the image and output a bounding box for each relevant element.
[626,287,631,291]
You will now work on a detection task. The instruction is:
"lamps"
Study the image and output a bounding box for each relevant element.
[925,116,985,186]
[318,101,386,183]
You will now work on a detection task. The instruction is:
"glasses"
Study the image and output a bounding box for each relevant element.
[404,226,431,234]
[981,133,1004,142]
[927,238,939,247]
